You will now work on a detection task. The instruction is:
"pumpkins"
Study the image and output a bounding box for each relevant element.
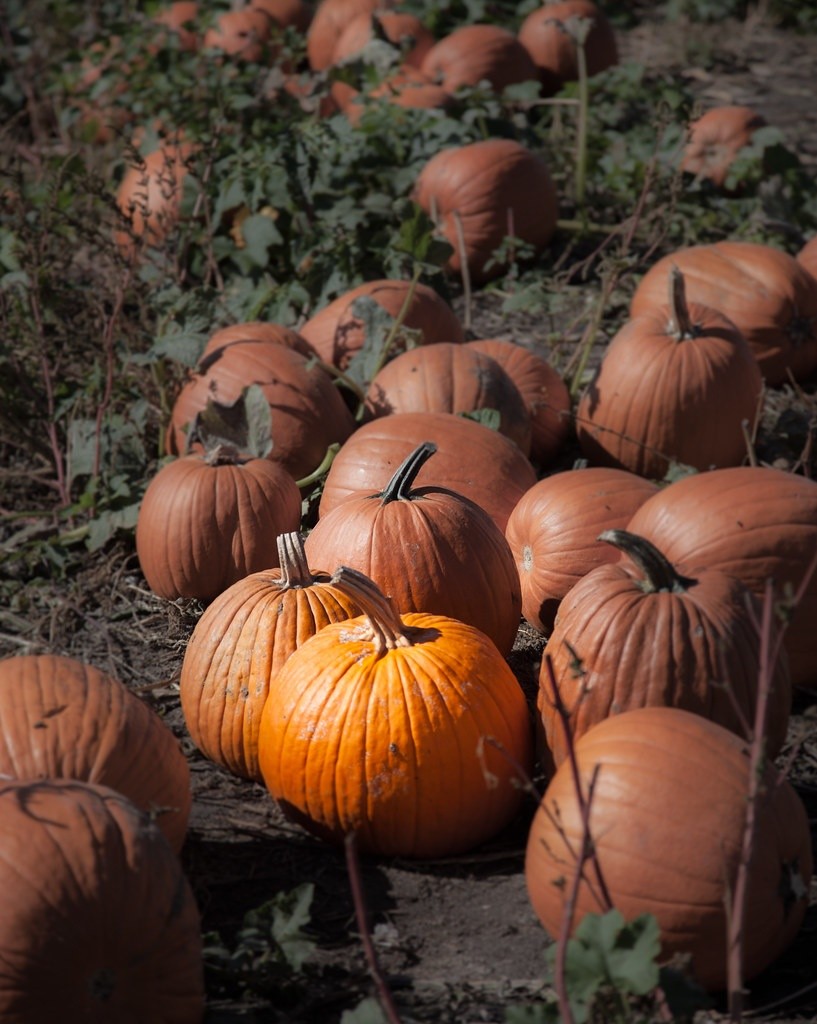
[0,0,817,1024]
